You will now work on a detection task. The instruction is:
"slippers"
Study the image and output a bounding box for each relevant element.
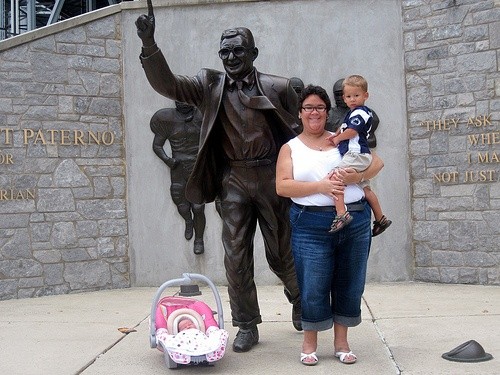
[335,348,358,363]
[300,352,318,365]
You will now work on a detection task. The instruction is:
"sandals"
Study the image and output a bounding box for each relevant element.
[329,211,354,233]
[371,215,392,237]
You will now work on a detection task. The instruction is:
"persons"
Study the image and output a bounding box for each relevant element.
[178,319,196,333]
[135,0,305,353]
[325,74,393,235]
[274,84,384,365]
[325,78,380,148]
[150,99,205,255]
[289,77,305,95]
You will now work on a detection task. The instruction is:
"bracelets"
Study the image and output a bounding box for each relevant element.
[356,172,364,184]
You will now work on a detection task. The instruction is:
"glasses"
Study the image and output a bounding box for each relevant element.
[300,106,327,112]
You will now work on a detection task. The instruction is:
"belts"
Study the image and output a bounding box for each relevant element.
[294,203,365,213]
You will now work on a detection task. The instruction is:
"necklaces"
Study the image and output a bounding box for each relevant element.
[301,133,326,151]
